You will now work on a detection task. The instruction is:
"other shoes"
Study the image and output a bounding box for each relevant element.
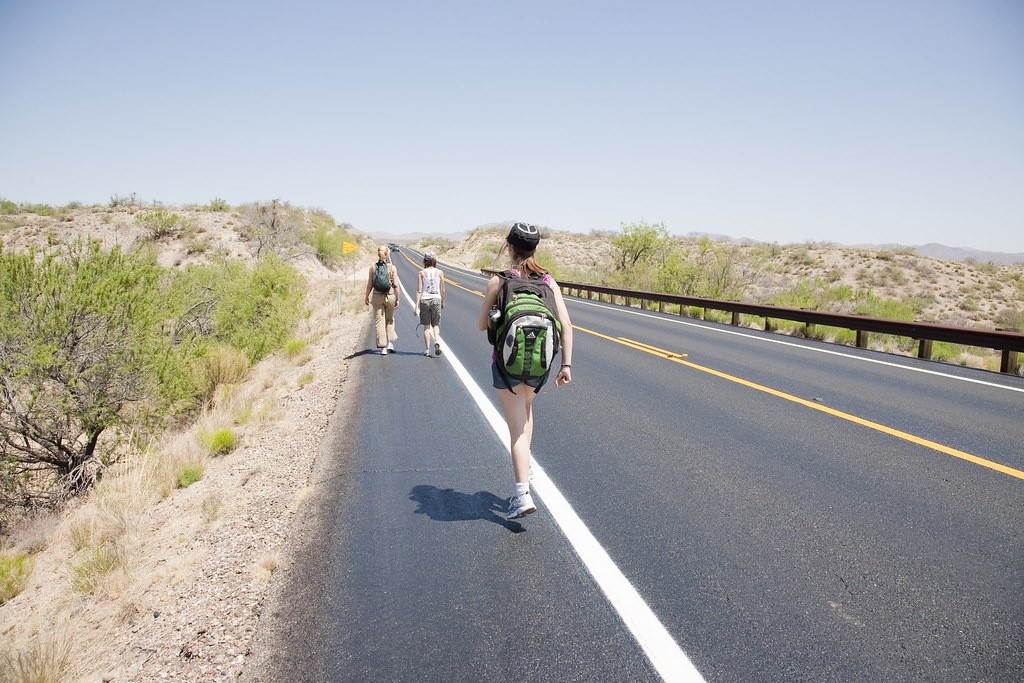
[423,351,431,356]
[434,342,441,355]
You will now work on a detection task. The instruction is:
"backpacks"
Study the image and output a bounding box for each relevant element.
[373,262,392,292]
[494,270,562,394]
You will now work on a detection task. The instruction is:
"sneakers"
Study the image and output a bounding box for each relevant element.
[380,348,387,355]
[527,465,535,481]
[387,346,396,353]
[507,491,536,519]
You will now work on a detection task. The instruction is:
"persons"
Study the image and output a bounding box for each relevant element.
[365,246,399,355]
[414,254,445,357]
[480,222,573,519]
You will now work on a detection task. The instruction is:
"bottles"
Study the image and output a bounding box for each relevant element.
[488,304,502,344]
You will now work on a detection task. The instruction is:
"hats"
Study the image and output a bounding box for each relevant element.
[424,251,435,260]
[505,222,540,250]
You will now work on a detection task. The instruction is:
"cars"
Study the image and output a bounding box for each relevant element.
[389,244,395,250]
[392,246,399,252]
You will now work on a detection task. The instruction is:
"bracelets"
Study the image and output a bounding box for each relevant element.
[561,365,572,367]
[396,299,399,301]
